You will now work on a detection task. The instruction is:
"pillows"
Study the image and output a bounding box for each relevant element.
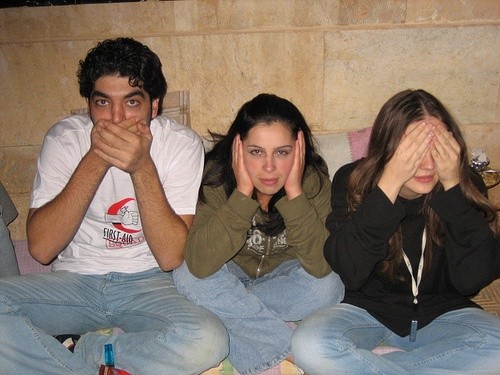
[311,127,373,183]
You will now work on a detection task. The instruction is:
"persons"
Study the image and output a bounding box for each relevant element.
[173,92,346,375]
[0,37,230,375]
[291,89,499,374]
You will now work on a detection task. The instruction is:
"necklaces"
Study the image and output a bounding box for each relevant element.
[401,224,428,341]
